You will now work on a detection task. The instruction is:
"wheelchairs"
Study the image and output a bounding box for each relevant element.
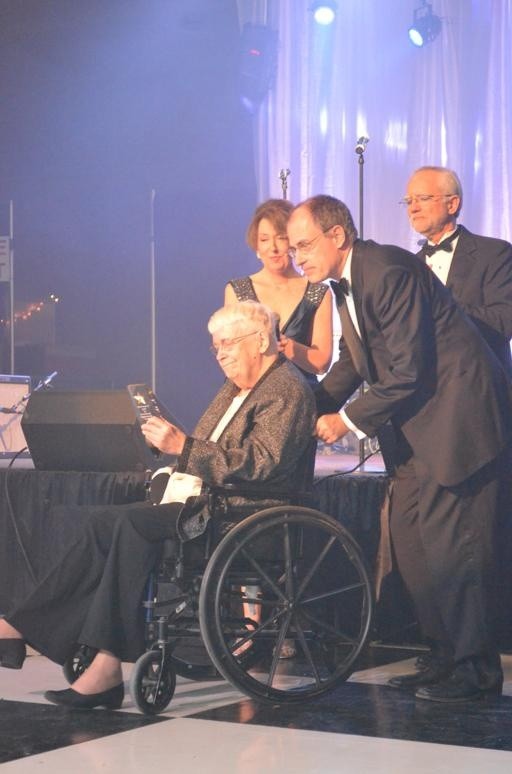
[64,441,375,714]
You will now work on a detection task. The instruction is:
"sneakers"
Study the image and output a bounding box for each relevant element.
[271,624,301,660]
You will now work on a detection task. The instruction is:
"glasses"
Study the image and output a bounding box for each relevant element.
[208,328,262,358]
[284,224,337,260]
[400,189,453,209]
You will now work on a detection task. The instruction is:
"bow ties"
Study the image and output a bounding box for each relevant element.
[422,242,454,258]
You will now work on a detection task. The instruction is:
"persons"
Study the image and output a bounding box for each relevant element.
[399,165,511,672]
[283,194,511,701]
[0,298,317,711]
[220,197,335,661]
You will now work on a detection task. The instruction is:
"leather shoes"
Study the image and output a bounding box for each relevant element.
[43,680,128,713]
[384,652,459,692]
[0,636,29,670]
[415,652,506,704]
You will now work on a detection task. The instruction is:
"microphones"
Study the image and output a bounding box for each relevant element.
[34,371,58,392]
[273,313,281,342]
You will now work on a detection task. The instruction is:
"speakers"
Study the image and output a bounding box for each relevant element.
[19,389,193,473]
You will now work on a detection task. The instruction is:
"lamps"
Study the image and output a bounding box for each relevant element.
[310,0,337,25]
[408,0,441,47]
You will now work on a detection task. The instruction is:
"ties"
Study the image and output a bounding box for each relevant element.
[329,276,372,383]
